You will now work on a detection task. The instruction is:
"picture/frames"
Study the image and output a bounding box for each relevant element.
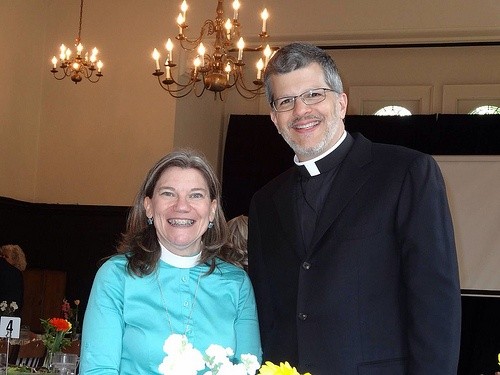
[346,85,433,116]
[442,83,500,115]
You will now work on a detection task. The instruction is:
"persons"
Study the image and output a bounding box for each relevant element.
[78,149,263,375]
[215,41,462,375]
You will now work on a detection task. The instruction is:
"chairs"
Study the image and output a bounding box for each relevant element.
[0,339,14,367]
[15,340,47,368]
[57,339,82,373]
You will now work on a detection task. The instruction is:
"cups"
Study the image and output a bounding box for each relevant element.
[0,352,7,375]
[53,354,77,375]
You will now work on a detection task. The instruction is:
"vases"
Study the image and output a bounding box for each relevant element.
[69,321,79,340]
[45,349,62,373]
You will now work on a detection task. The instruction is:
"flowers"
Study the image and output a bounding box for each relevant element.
[37,317,74,353]
[0,300,18,317]
[60,296,80,327]
[157,333,312,375]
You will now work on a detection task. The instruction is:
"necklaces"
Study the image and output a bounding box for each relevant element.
[150,255,204,346]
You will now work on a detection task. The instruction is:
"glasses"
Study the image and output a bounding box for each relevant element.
[270,87,342,112]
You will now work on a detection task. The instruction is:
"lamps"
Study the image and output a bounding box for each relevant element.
[50,0,104,85]
[151,0,277,103]
